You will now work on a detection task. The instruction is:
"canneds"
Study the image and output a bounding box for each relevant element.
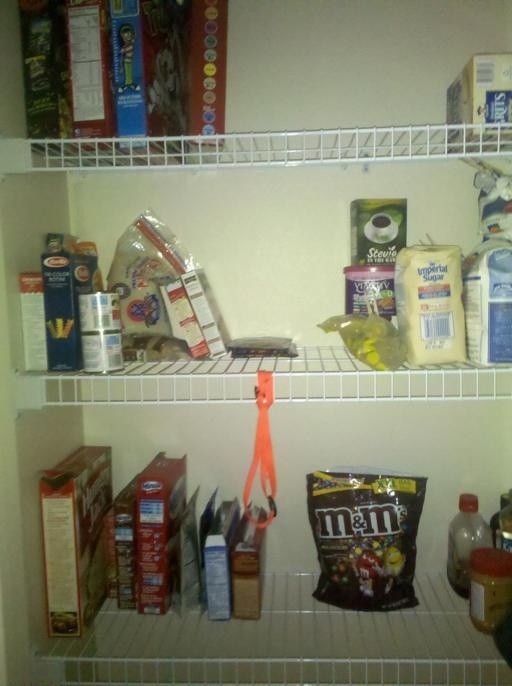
[343,265,395,321]
[76,292,125,375]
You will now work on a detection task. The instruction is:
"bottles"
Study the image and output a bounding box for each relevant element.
[342,264,398,335]
[489,487,511,553]
[445,494,494,598]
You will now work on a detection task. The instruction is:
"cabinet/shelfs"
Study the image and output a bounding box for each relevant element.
[0,0,510,686]
